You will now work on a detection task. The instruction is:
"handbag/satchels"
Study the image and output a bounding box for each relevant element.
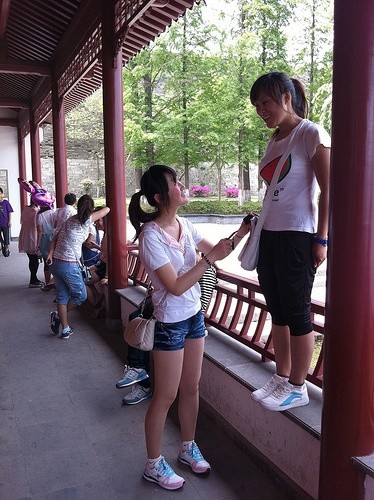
[238,217,259,271]
[124,316,155,350]
[81,266,93,284]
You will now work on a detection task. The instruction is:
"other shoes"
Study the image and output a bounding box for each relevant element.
[29,282,42,288]
[44,282,56,289]
[3,250,9,256]
[94,294,105,308]
[38,280,44,285]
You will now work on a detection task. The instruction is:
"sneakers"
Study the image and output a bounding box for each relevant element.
[251,373,286,402]
[142,456,186,490]
[116,365,150,389]
[122,384,152,405]
[62,327,74,339]
[260,378,309,411]
[50,311,61,335]
[178,441,211,474]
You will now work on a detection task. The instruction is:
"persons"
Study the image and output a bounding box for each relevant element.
[248,71,332,416]
[20,195,45,288]
[0,187,12,257]
[129,166,261,492]
[114,248,218,405]
[45,194,111,338]
[36,192,108,320]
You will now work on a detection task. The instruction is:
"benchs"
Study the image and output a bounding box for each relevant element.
[116,251,374,500]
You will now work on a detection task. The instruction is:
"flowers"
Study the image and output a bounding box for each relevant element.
[224,187,238,198]
[189,185,211,196]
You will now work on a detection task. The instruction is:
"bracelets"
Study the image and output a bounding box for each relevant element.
[316,236,328,244]
[236,232,243,238]
[200,255,215,270]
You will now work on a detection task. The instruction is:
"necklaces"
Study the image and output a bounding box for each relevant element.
[274,119,302,142]
